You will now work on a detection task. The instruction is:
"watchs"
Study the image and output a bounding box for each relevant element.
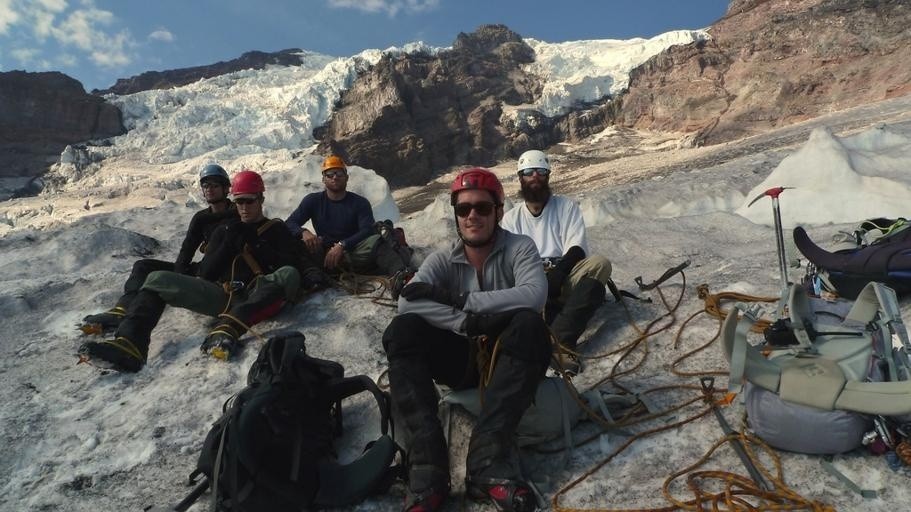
[338,241,346,247]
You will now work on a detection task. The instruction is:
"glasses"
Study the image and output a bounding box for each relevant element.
[202,181,221,188]
[236,198,257,205]
[455,201,496,217]
[325,171,343,178]
[523,168,546,176]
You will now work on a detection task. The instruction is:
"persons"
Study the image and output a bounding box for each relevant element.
[286,156,377,291]
[499,149,611,376]
[81,171,303,372]
[382,168,553,512]
[84,166,241,331]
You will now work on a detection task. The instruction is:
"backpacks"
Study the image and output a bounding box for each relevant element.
[720,281,911,454]
[174,332,405,512]
[794,218,911,300]
[356,219,413,275]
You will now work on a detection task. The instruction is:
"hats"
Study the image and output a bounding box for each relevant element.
[233,194,258,201]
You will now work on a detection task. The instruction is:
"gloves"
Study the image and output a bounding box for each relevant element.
[547,262,569,298]
[400,282,470,311]
[460,307,533,337]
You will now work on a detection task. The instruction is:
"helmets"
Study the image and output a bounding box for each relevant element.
[200,165,229,180]
[517,150,551,174]
[231,171,265,195]
[321,156,345,175]
[451,169,504,206]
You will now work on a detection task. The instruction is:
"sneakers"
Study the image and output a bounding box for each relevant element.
[81,307,127,335]
[200,324,238,361]
[302,271,329,289]
[465,460,536,511]
[78,337,145,373]
[551,336,578,377]
[404,474,450,512]
[390,268,417,298]
